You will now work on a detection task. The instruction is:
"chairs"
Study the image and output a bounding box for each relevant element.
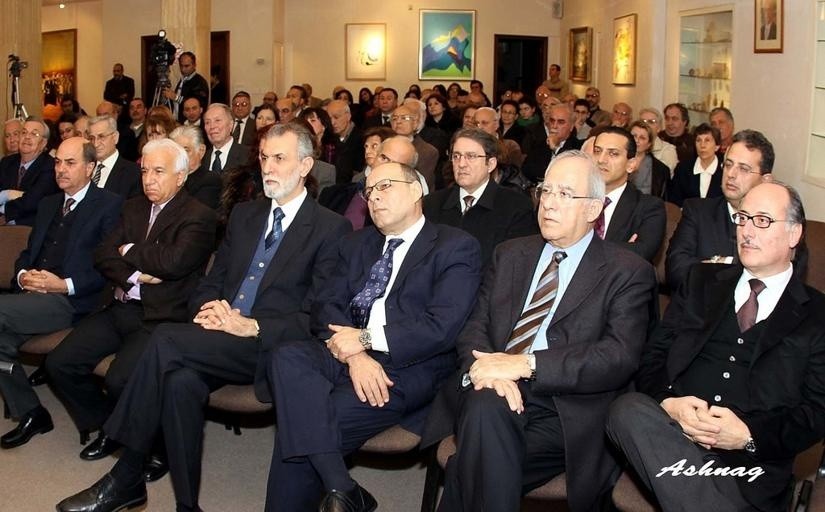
[2,160,825,511]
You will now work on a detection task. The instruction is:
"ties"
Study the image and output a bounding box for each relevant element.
[736,278,767,335]
[143,204,162,242]
[349,237,405,330]
[211,149,223,173]
[502,249,569,356]
[732,213,738,266]
[462,194,476,216]
[264,205,286,251]
[13,165,26,191]
[593,196,613,242]
[62,197,77,218]
[231,119,245,145]
[91,163,105,187]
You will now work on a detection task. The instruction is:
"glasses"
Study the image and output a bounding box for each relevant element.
[463,92,658,128]
[389,115,415,123]
[720,160,763,175]
[275,108,294,115]
[362,178,413,198]
[233,102,250,108]
[731,212,794,229]
[18,129,46,139]
[533,181,596,201]
[88,131,115,144]
[262,97,276,102]
[450,151,491,159]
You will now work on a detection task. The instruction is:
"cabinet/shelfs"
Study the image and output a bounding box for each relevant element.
[677,8,738,116]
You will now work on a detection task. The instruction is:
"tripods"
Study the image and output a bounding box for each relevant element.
[12,77,28,118]
[151,86,172,110]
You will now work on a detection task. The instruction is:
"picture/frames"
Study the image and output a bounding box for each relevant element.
[567,26,592,83]
[612,12,638,86]
[752,0,784,55]
[42,28,77,123]
[345,23,387,81]
[418,8,476,83]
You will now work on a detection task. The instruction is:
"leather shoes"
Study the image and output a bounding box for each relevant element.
[0,406,55,450]
[142,454,171,483]
[79,431,126,461]
[27,365,49,388]
[55,472,149,512]
[316,477,379,512]
